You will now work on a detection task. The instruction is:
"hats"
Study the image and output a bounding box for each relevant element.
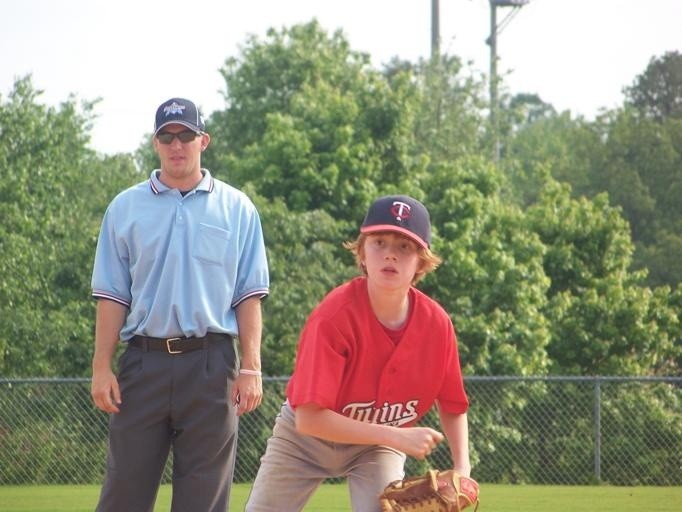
[153,98,206,137]
[359,195,432,251]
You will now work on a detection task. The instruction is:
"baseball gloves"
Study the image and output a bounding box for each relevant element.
[381,470,481,512]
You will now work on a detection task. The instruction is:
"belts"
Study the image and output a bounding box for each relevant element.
[127,332,229,354]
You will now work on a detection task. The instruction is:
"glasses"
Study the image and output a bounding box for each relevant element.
[156,129,196,144]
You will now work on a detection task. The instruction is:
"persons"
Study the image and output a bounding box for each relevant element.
[244,194,481,512]
[91,97,270,511]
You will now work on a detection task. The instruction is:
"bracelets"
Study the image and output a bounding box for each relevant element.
[238,367,262,378]
